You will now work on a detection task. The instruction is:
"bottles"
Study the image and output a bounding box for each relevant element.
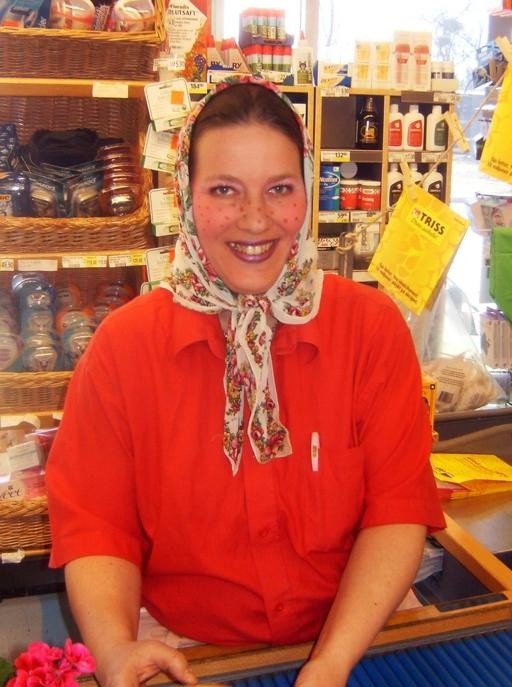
[389,104,448,211]
[356,97,380,149]
[356,43,391,81]
[208,7,313,85]
[396,43,429,85]
[431,61,455,79]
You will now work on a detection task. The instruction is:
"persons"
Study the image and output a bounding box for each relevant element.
[44,77,446,687]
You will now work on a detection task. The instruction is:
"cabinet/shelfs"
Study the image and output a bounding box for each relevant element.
[212,6,458,364]
[0,0,211,572]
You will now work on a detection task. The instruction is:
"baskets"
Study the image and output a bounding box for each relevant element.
[0,266,144,412]
[0,95,154,254]
[0,416,52,549]
[0,0,169,81]
